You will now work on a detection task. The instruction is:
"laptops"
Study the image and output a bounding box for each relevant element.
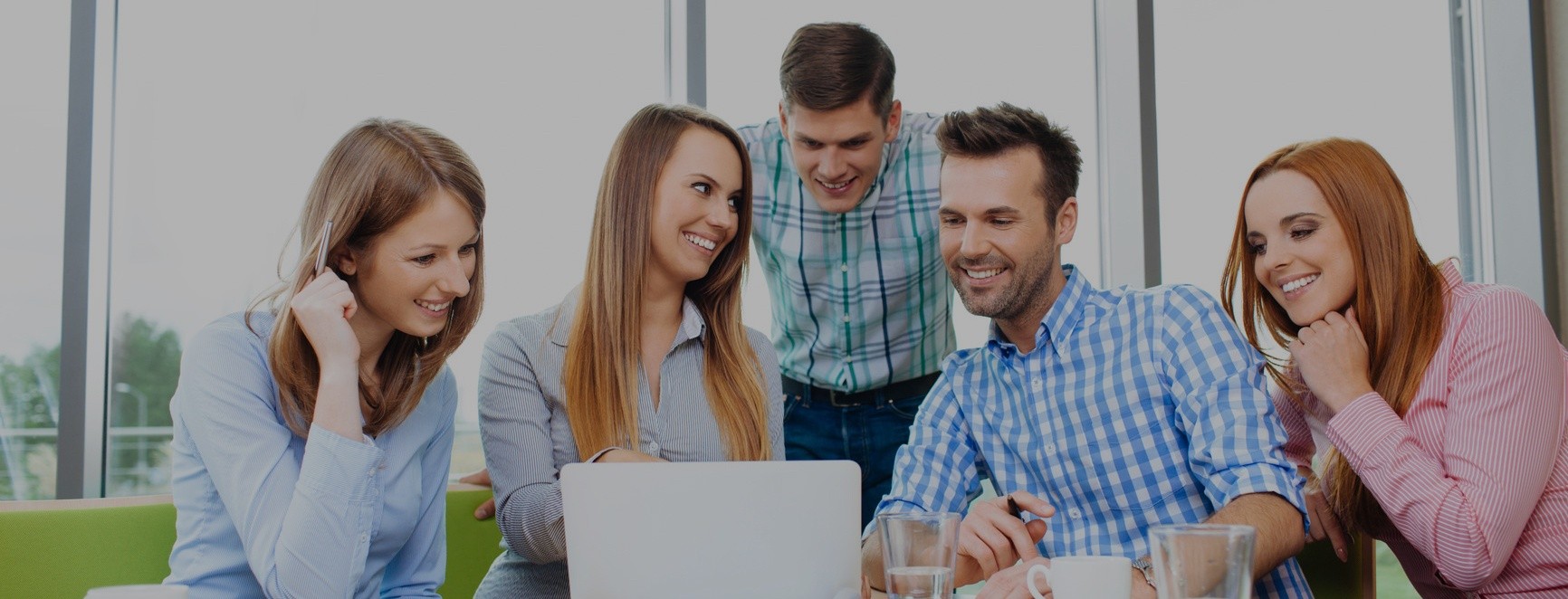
[560,461,864,598]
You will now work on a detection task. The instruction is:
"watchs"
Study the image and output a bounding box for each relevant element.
[1132,558,1157,590]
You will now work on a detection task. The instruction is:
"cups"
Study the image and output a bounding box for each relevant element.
[1025,555,1133,599]
[875,509,963,599]
[1145,524,1259,598]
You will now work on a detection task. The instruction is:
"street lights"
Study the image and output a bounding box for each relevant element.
[116,384,145,496]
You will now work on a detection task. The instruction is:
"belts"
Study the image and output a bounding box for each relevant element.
[781,370,943,408]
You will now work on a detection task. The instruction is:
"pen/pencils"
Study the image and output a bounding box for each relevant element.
[1007,494,1026,524]
[315,219,333,279]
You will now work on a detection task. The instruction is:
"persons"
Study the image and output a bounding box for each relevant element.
[1221,138,1568,599]
[161,118,486,599]
[861,97,1312,599]
[458,22,955,532]
[473,102,788,599]
